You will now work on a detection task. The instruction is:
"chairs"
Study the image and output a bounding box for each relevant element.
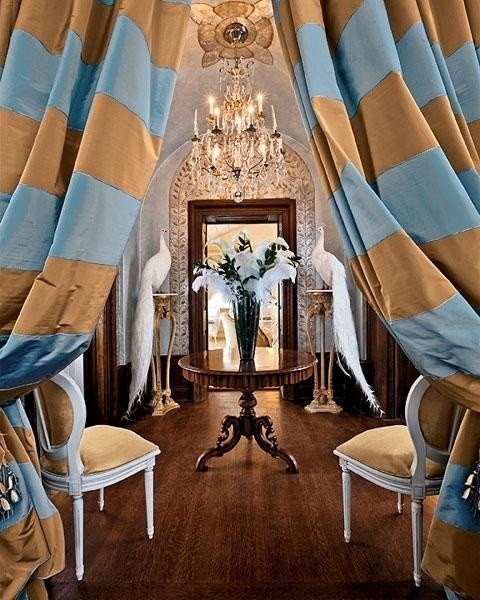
[18,369,161,581]
[334,374,466,588]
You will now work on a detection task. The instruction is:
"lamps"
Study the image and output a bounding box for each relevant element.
[188,53,286,203]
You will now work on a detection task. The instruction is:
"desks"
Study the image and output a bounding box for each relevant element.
[178,347,318,474]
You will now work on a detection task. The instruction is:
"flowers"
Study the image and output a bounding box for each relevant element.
[191,230,300,304]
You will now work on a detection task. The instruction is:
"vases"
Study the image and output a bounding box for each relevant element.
[232,299,260,361]
